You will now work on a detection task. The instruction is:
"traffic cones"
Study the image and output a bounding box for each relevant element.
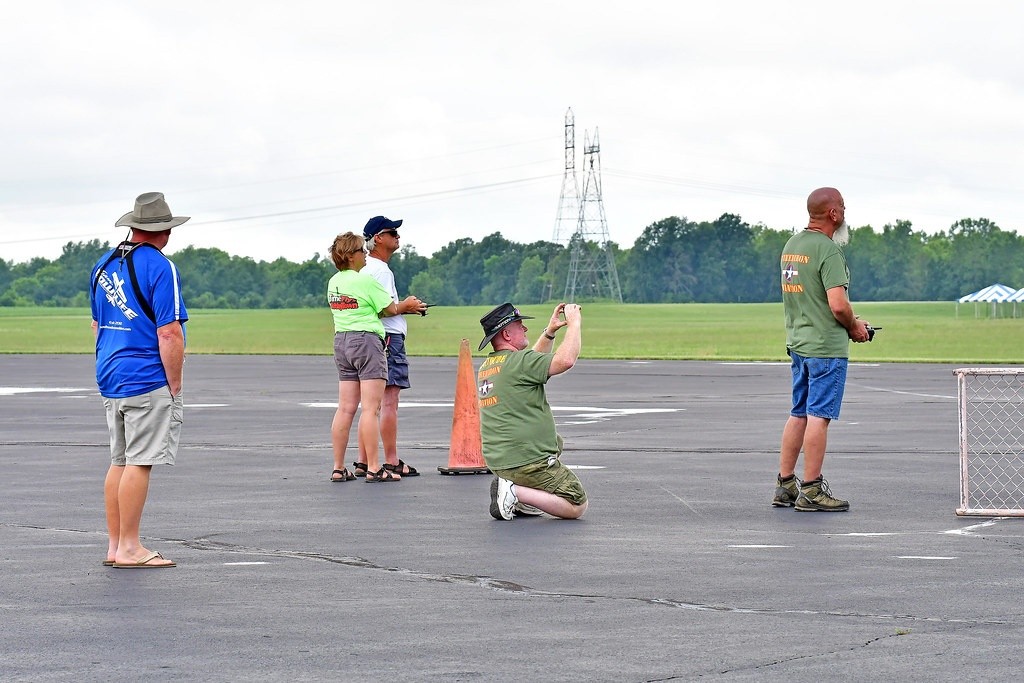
[435,341,492,475]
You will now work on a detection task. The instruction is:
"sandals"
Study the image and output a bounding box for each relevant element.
[365,466,401,483]
[331,468,358,482]
[353,462,369,477]
[382,459,420,476]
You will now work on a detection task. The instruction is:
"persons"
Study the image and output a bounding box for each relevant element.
[479,304,588,518]
[90,193,191,568]
[353,215,429,476]
[327,232,402,481]
[773,185,872,512]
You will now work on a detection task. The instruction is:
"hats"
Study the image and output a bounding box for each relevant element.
[115,192,191,232]
[477,303,535,351]
[363,216,403,242]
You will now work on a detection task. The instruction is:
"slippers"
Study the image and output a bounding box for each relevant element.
[103,549,177,568]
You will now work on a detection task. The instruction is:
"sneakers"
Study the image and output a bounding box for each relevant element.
[771,473,801,507]
[795,474,850,512]
[490,477,517,521]
[513,501,545,517]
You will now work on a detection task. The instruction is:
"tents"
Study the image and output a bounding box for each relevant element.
[1006,286,1024,319]
[955,281,1018,319]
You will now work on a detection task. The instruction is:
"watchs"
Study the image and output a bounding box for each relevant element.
[542,327,555,340]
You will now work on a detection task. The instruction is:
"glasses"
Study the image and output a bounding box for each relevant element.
[352,246,363,254]
[497,308,520,323]
[379,231,398,238]
